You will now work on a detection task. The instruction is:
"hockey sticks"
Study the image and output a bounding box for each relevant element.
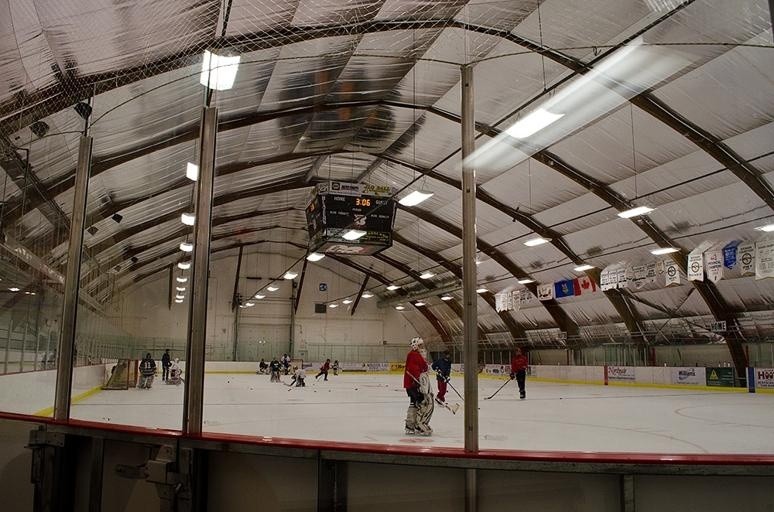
[284,380,296,387]
[482,378,511,400]
[445,376,481,410]
[403,370,459,415]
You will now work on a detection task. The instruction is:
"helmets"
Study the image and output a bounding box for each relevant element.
[409,336,425,352]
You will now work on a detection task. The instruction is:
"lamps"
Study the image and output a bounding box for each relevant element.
[236,189,433,311]
[26,96,138,274]
[329,203,680,311]
[176,50,242,303]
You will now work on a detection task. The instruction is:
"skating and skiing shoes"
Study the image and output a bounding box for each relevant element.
[403,421,434,437]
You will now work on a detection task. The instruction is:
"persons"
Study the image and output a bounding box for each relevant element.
[510,346,530,398]
[168,359,183,385]
[159,348,171,382]
[135,352,157,390]
[256,353,341,388]
[432,350,451,404]
[403,338,435,436]
